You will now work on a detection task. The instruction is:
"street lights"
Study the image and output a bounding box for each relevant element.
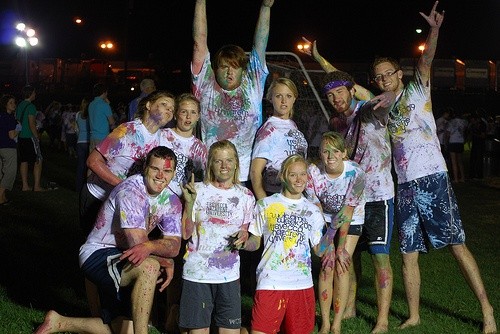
[16,23,38,85]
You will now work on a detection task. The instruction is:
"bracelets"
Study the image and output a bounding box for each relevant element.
[262,3,272,7]
[330,224,338,231]
[241,242,247,249]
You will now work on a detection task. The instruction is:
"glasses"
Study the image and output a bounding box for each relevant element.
[373,70,400,82]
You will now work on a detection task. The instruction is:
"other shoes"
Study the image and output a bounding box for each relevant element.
[1,202,8,207]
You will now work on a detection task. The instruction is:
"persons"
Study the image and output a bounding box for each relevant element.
[299,0,498,333]
[323,71,397,333]
[190,0,276,187]
[149,93,210,333]
[0,95,23,208]
[226,155,356,334]
[78,91,176,317]
[250,78,309,201]
[34,146,185,333]
[0,48,500,192]
[178,139,256,333]
[304,131,368,333]
